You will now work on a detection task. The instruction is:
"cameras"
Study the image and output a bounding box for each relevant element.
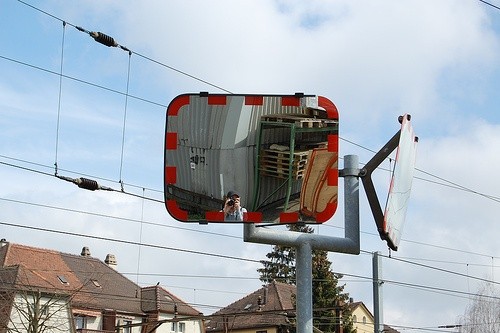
[228,198,235,206]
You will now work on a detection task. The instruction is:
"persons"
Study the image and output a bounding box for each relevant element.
[219,191,247,222]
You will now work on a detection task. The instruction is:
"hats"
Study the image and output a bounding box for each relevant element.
[226,190,240,198]
